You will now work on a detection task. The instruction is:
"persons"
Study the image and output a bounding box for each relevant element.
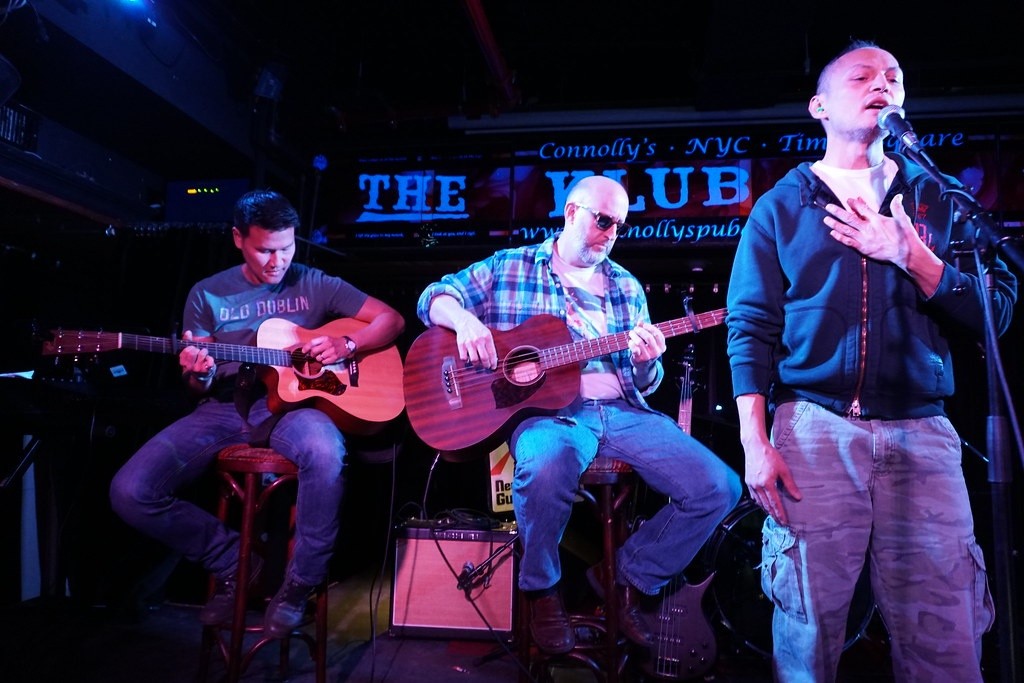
[415,174,741,653]
[108,189,404,635]
[726,41,1017,683]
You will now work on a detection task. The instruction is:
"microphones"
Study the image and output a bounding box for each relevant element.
[878,105,923,154]
[458,564,474,583]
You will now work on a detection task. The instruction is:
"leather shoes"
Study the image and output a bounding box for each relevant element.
[585,560,657,647]
[263,559,315,640]
[522,586,576,653]
[199,552,263,625]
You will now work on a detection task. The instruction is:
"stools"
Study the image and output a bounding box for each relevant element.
[196,444,329,683]
[517,456,642,683]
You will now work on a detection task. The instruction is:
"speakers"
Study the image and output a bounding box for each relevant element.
[388,526,515,643]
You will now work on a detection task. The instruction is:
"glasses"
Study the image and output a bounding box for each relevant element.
[576,203,631,235]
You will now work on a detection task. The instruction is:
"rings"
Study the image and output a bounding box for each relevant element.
[482,360,489,363]
[206,366,209,369]
[320,354,325,360]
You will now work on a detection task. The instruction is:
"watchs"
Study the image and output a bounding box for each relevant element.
[343,336,357,358]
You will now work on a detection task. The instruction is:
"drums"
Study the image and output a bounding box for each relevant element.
[704,495,878,660]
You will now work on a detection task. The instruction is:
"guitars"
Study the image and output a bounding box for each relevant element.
[402,308,729,465]
[41,316,406,438]
[629,295,717,683]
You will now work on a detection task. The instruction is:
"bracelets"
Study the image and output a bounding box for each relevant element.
[198,365,216,382]
